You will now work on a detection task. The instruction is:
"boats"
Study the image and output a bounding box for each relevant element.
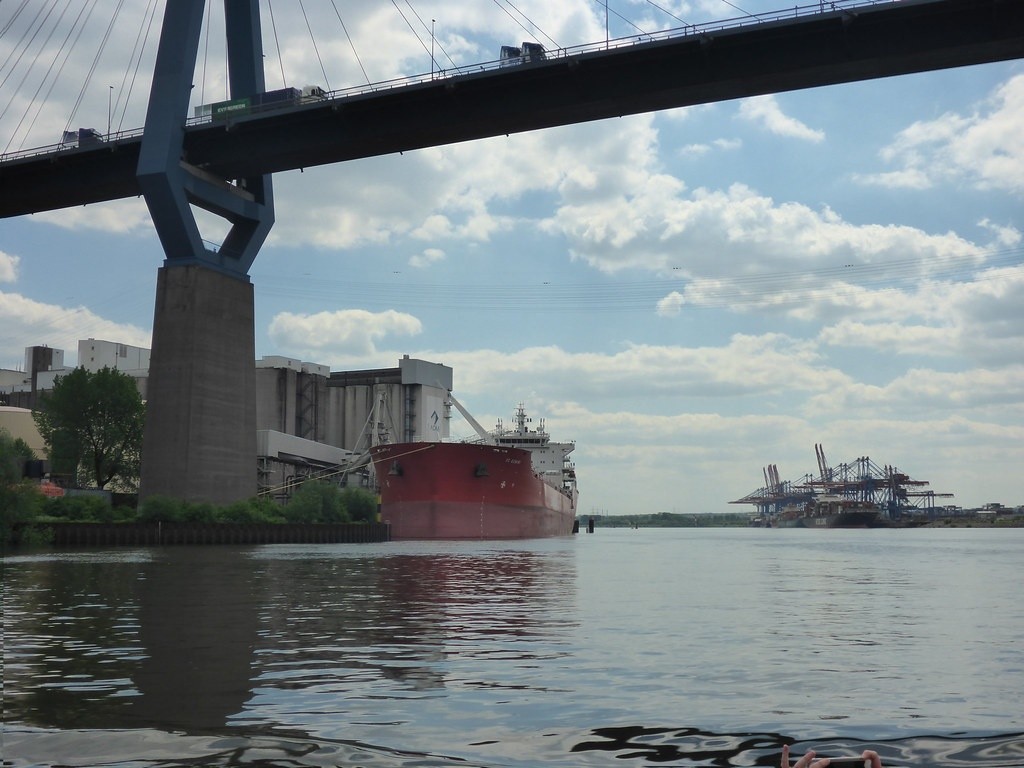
[802,511,881,529]
[366,377,579,542]
